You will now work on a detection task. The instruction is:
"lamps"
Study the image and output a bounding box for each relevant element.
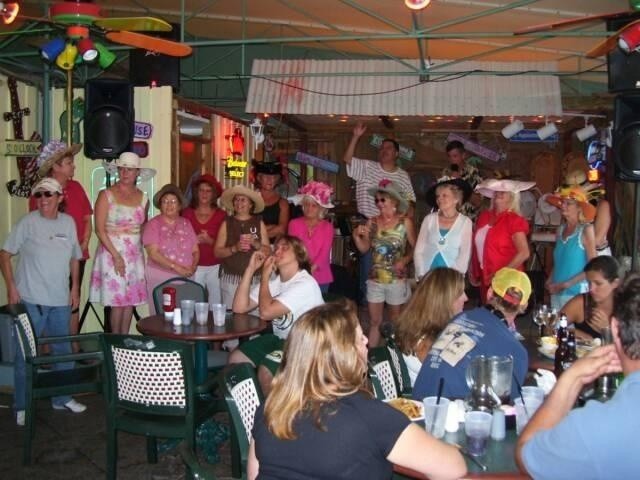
[403,0,432,11]
[1,0,19,25]
[501,113,607,142]
[617,22,640,54]
[40,25,118,71]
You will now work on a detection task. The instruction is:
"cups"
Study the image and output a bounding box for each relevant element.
[182,325,194,336]
[422,396,450,439]
[195,302,210,325]
[514,397,542,436]
[195,325,208,335]
[211,303,227,327]
[180,299,196,326]
[239,234,251,250]
[520,385,545,405]
[464,411,492,456]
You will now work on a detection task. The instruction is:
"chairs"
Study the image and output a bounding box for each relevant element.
[360,346,402,419]
[384,341,412,393]
[153,277,207,318]
[12,314,110,466]
[220,361,265,480]
[99,333,217,479]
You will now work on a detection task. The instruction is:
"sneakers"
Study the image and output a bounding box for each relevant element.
[16,410,25,426]
[52,399,87,413]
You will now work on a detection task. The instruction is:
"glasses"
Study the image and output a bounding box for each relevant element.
[161,199,178,204]
[374,198,385,202]
[34,191,53,198]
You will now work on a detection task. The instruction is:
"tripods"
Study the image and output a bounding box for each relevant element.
[76,171,141,334]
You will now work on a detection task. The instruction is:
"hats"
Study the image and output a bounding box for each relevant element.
[491,267,532,305]
[546,187,596,223]
[368,179,408,211]
[153,184,187,210]
[426,175,472,207]
[191,174,223,197]
[287,181,335,209]
[102,152,156,179]
[553,171,602,193]
[475,179,536,198]
[222,185,264,213]
[32,178,63,197]
[38,140,82,176]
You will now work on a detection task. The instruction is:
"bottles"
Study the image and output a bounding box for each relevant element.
[556,311,568,345]
[489,409,507,441]
[172,325,182,335]
[562,330,585,407]
[554,331,567,379]
[173,308,182,326]
[594,327,625,403]
[162,287,177,318]
[444,401,458,433]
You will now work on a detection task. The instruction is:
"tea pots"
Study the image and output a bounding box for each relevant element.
[465,354,514,405]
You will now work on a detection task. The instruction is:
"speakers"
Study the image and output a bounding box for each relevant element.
[83,78,135,160]
[607,12,640,93]
[129,22,181,95]
[613,92,640,182]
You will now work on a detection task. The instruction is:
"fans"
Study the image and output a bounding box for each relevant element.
[514,0,640,59]
[0,0,194,74]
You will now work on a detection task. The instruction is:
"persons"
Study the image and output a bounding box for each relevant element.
[2,119,638,400]
[248,300,466,480]
[515,270,640,479]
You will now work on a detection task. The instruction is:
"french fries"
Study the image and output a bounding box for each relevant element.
[387,397,419,418]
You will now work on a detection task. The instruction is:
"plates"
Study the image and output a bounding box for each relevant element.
[537,345,583,360]
[536,336,592,350]
[381,399,426,421]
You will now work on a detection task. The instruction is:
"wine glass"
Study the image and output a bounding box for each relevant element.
[532,303,547,345]
[543,307,558,337]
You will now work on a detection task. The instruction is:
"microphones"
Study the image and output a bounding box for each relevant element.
[451,163,459,171]
